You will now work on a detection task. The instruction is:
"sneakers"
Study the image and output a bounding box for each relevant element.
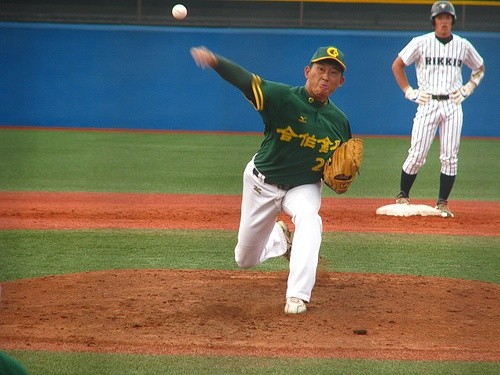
[278,220,292,261]
[284,297,307,315]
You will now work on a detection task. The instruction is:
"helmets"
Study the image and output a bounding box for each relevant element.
[429,0,457,25]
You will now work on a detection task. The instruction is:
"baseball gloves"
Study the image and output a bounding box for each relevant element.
[322,137,364,195]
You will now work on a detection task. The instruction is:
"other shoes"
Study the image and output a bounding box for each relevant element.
[396,197,410,205]
[435,197,450,217]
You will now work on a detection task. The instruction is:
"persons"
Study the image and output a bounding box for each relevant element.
[391,0,486,218]
[189,45,352,315]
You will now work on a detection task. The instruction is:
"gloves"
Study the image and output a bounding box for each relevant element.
[450,84,473,104]
[403,86,433,105]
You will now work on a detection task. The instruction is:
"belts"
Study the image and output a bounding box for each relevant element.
[432,95,449,99]
[253,168,304,191]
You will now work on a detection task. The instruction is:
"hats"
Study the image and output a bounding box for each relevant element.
[310,46,346,73]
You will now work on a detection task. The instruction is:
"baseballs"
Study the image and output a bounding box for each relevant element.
[172,4,188,20]
